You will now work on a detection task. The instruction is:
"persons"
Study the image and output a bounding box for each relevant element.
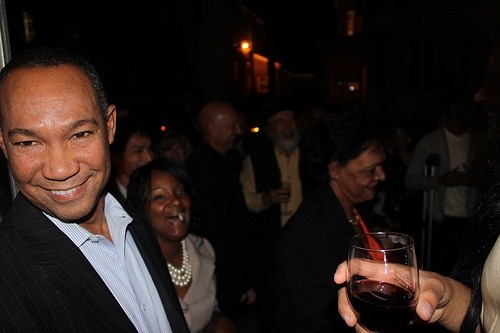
[0,47,191,333]
[109,100,500,333]
[333,46,500,333]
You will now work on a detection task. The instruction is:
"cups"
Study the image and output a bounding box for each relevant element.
[346,232,420,333]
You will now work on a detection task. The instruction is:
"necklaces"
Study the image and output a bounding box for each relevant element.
[166,239,192,287]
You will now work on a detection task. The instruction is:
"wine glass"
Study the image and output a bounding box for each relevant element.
[279,181,293,217]
